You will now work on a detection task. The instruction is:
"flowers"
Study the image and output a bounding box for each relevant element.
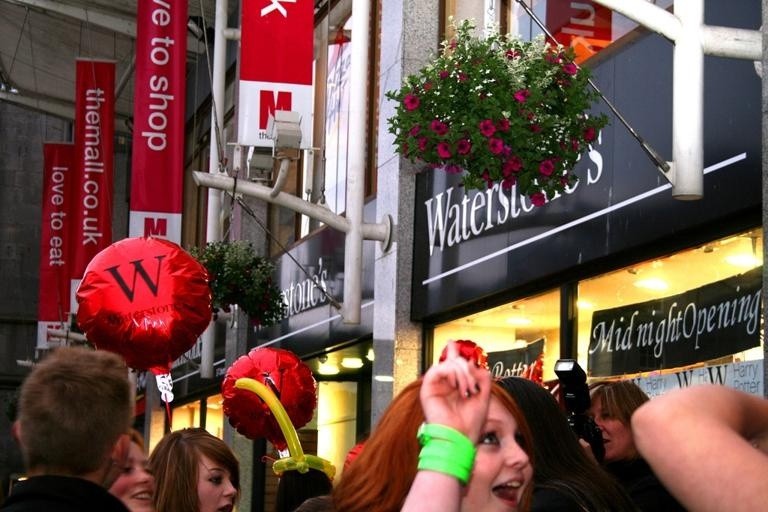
[189,241,288,329]
[386,12,611,208]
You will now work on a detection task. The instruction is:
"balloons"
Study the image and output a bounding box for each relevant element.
[71,237,214,376]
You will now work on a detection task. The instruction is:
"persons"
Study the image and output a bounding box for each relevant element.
[1,337,767,512]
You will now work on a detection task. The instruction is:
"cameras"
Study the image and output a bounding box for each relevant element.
[554,359,606,465]
